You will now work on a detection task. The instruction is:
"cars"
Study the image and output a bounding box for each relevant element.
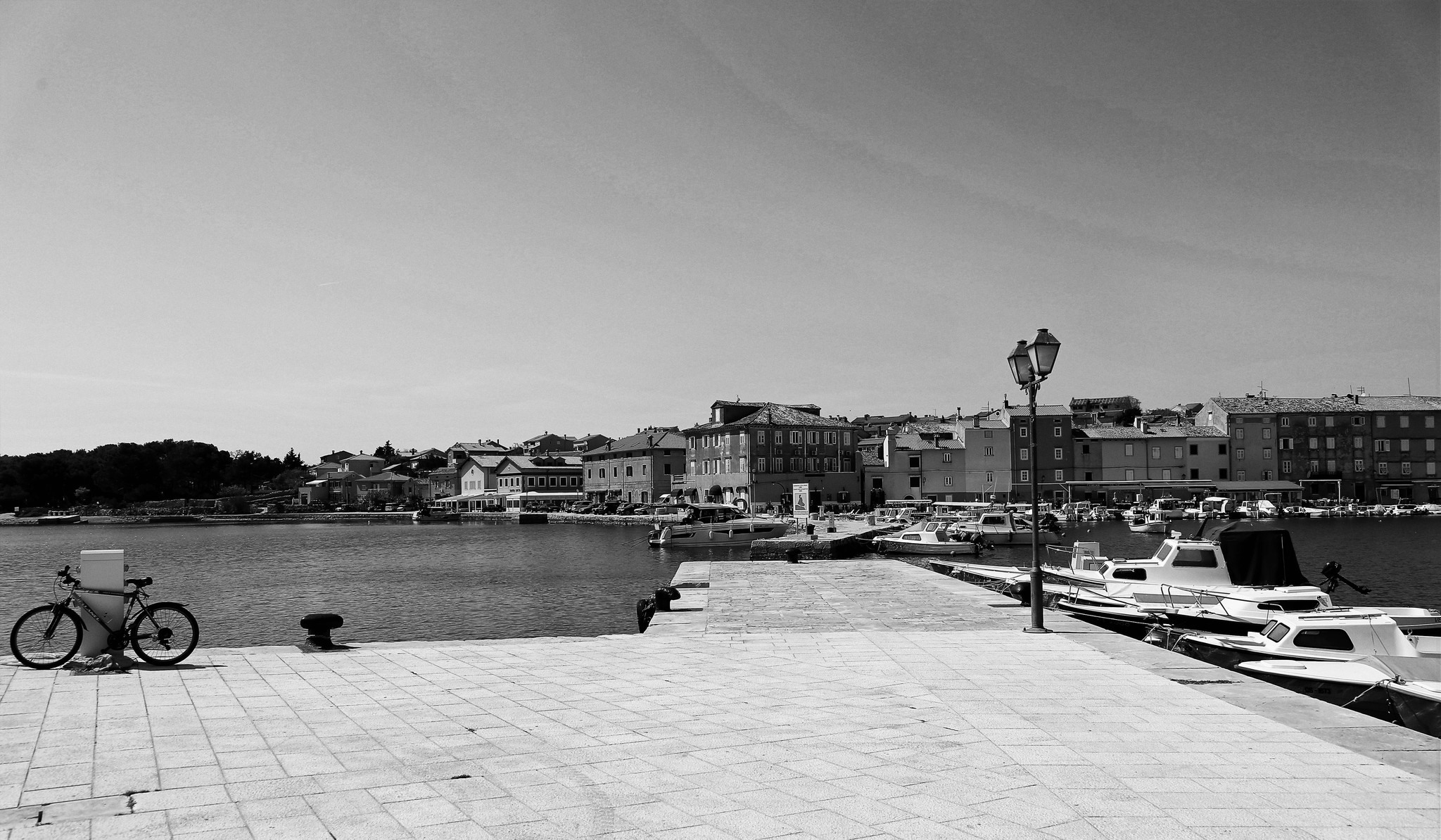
[482,504,504,513]
[564,500,660,515]
[367,503,410,512]
[539,503,561,513]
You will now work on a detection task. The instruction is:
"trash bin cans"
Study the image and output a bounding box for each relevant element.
[805,524,815,535]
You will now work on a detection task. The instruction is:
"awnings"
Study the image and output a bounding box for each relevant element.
[736,486,748,493]
[585,488,622,493]
[685,488,697,496]
[670,488,683,497]
[723,487,732,493]
[709,484,722,496]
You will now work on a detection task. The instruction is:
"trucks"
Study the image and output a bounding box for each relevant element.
[655,494,672,504]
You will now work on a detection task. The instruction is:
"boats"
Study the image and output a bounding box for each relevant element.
[833,492,1441,740]
[411,506,462,521]
[647,503,796,548]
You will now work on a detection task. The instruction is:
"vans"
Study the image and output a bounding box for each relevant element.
[341,504,354,512]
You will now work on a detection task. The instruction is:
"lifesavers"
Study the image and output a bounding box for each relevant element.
[733,498,748,512]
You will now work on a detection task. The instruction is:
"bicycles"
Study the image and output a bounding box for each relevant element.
[9,565,200,668]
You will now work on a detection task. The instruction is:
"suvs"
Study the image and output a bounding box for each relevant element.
[525,502,541,513]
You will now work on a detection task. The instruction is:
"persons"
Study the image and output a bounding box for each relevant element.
[560,502,569,513]
[1112,498,1152,510]
[684,505,693,525]
[1194,494,1198,510]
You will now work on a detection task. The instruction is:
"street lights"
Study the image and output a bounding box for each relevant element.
[651,486,655,503]
[525,491,528,504]
[576,489,579,500]
[1006,327,1063,634]
